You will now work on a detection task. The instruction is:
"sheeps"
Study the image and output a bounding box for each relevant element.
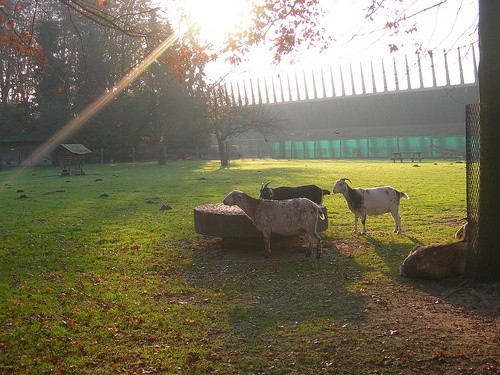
[259,181,331,205]
[333,178,409,236]
[400,217,479,281]
[223,189,326,260]
